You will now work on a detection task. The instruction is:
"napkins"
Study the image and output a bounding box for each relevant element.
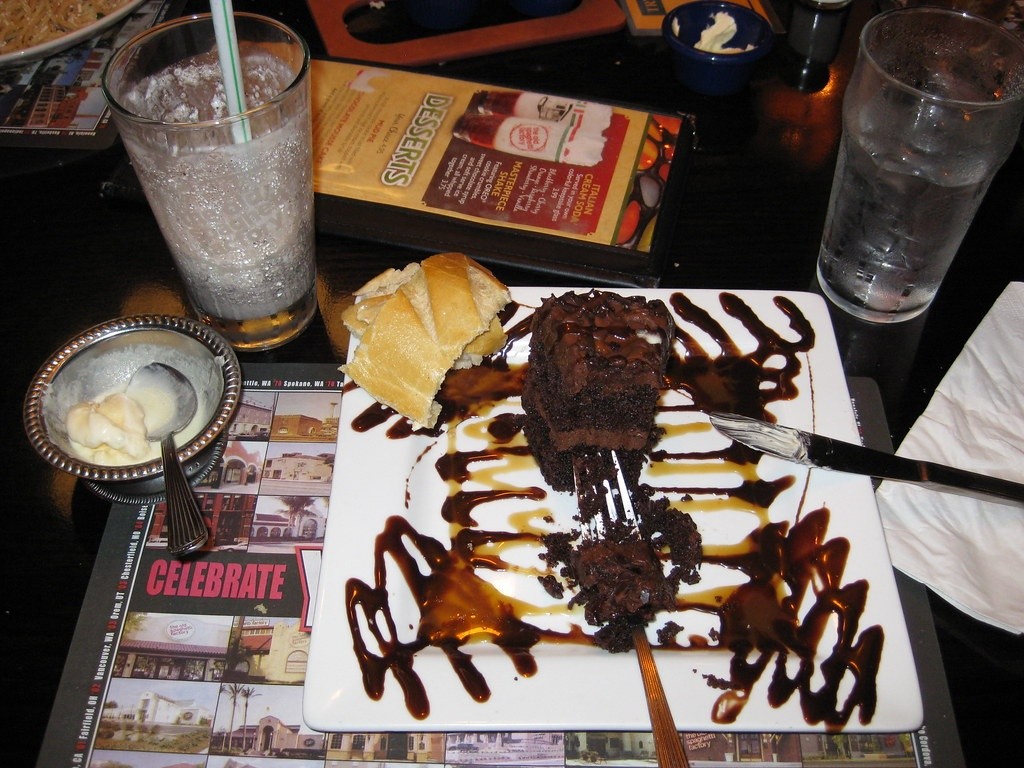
[875,282,1024,636]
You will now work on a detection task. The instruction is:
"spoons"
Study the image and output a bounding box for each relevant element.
[123,360,209,556]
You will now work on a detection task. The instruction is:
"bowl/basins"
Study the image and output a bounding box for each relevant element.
[21,314,242,506]
[662,0,774,98]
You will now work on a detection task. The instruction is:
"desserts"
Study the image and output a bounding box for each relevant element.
[522,289,673,490]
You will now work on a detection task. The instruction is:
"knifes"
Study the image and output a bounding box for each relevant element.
[709,409,1024,503]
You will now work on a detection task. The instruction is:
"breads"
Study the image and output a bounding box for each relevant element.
[341,253,511,425]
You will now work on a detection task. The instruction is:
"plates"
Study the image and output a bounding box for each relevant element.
[0,0,145,68]
[302,286,926,732]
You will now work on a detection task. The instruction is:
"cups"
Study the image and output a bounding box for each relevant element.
[816,8,1024,324]
[452,89,613,166]
[100,12,319,353]
[782,0,851,92]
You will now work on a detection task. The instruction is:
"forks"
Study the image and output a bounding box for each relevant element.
[571,451,691,768]
